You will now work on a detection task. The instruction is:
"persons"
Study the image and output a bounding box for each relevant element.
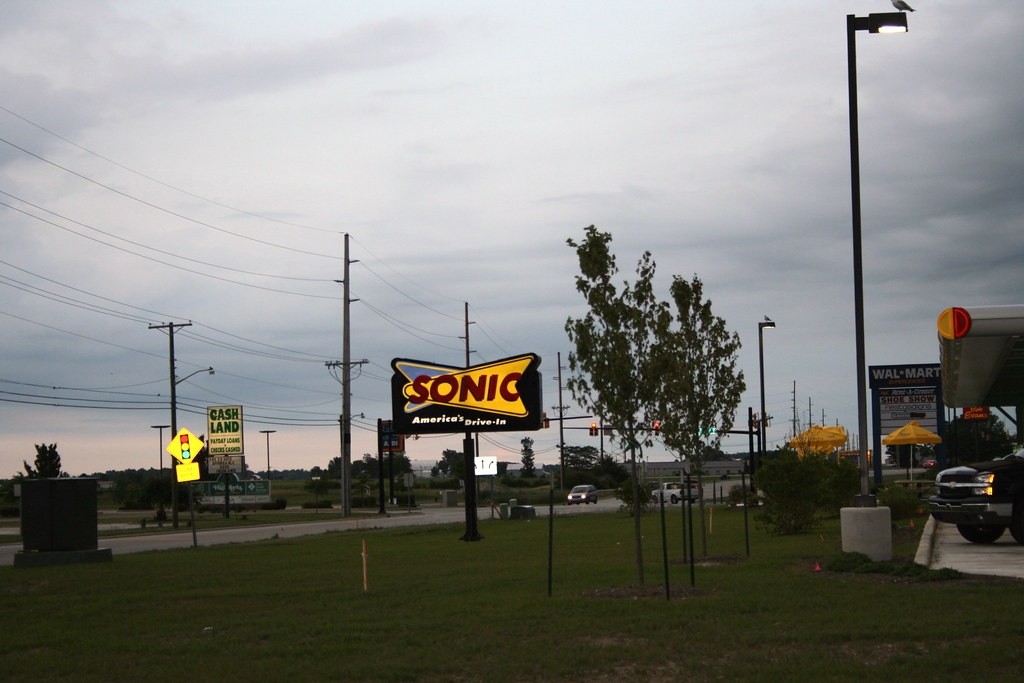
[157,504,167,527]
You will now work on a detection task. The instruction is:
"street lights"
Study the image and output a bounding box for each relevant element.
[340,412,366,518]
[258,430,276,500]
[170,365,215,530]
[758,319,779,457]
[151,424,170,522]
[846,11,909,508]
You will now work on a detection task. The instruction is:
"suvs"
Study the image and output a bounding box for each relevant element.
[925,446,1024,547]
[567,484,598,505]
[683,480,705,500]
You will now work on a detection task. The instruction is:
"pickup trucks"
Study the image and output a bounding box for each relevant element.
[651,481,686,504]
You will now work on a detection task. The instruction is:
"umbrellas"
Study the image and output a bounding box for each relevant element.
[790,425,847,465]
[882,420,942,482]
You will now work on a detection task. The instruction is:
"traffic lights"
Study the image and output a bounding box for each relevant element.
[595,429,598,436]
[655,422,660,436]
[751,413,757,427]
[180,433,191,459]
[590,428,593,436]
